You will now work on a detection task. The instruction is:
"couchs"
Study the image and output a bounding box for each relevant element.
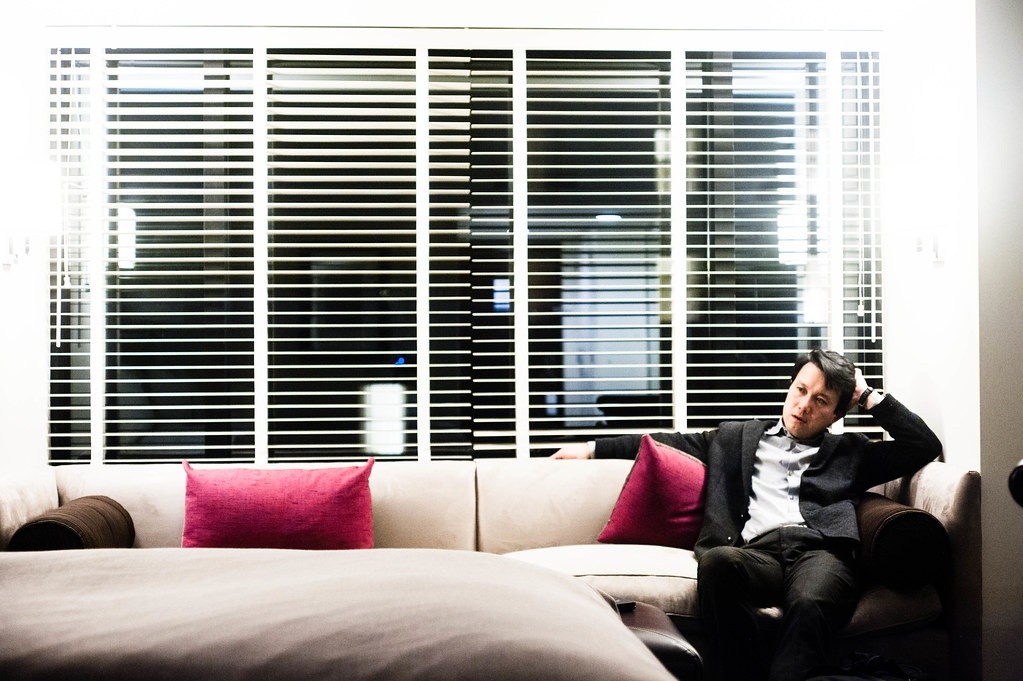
[0,456,986,681]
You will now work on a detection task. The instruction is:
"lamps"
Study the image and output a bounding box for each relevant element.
[115,205,136,273]
[365,383,406,458]
[775,195,812,268]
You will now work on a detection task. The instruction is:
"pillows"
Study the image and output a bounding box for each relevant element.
[852,488,954,596]
[7,494,136,551]
[179,459,375,550]
[596,432,711,551]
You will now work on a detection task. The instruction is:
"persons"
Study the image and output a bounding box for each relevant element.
[550,349,943,680]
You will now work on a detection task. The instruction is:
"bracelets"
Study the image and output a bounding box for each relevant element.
[856,387,873,408]
